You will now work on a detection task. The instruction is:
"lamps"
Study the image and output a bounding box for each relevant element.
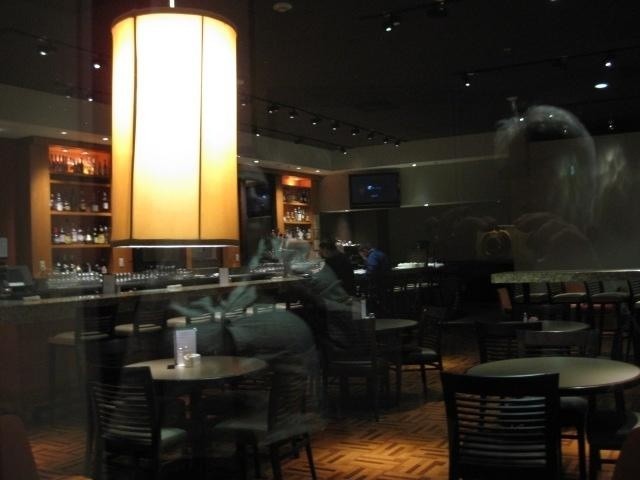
[240,93,406,155]
[108,0,240,250]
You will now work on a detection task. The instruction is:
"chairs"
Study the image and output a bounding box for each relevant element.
[50,274,331,478]
[441,372,578,478]
[332,274,639,426]
[466,356,639,479]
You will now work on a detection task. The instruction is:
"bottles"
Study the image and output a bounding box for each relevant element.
[284,206,310,222]
[50,187,110,213]
[286,226,312,241]
[52,222,110,245]
[50,154,108,175]
[249,261,320,272]
[283,189,310,202]
[34,260,192,290]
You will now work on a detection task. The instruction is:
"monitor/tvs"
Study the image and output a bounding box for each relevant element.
[348,172,400,208]
[245,183,274,220]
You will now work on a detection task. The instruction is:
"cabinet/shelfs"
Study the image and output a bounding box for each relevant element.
[17,135,111,275]
[320,155,512,269]
[273,174,314,245]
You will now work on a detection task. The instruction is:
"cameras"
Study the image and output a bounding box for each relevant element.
[475,223,531,258]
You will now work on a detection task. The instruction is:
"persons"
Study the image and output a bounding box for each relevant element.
[108,155,368,459]
[419,104,613,379]
